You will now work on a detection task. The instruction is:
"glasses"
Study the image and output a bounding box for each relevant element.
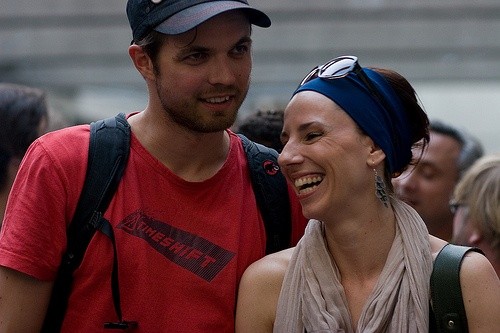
[299,55,401,134]
[450,199,469,213]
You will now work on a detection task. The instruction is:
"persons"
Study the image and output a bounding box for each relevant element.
[236,55,500,333]
[237,109,500,279]
[0,0,308,333]
[0,83,52,234]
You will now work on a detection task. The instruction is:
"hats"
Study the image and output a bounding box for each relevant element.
[126,0,271,45]
